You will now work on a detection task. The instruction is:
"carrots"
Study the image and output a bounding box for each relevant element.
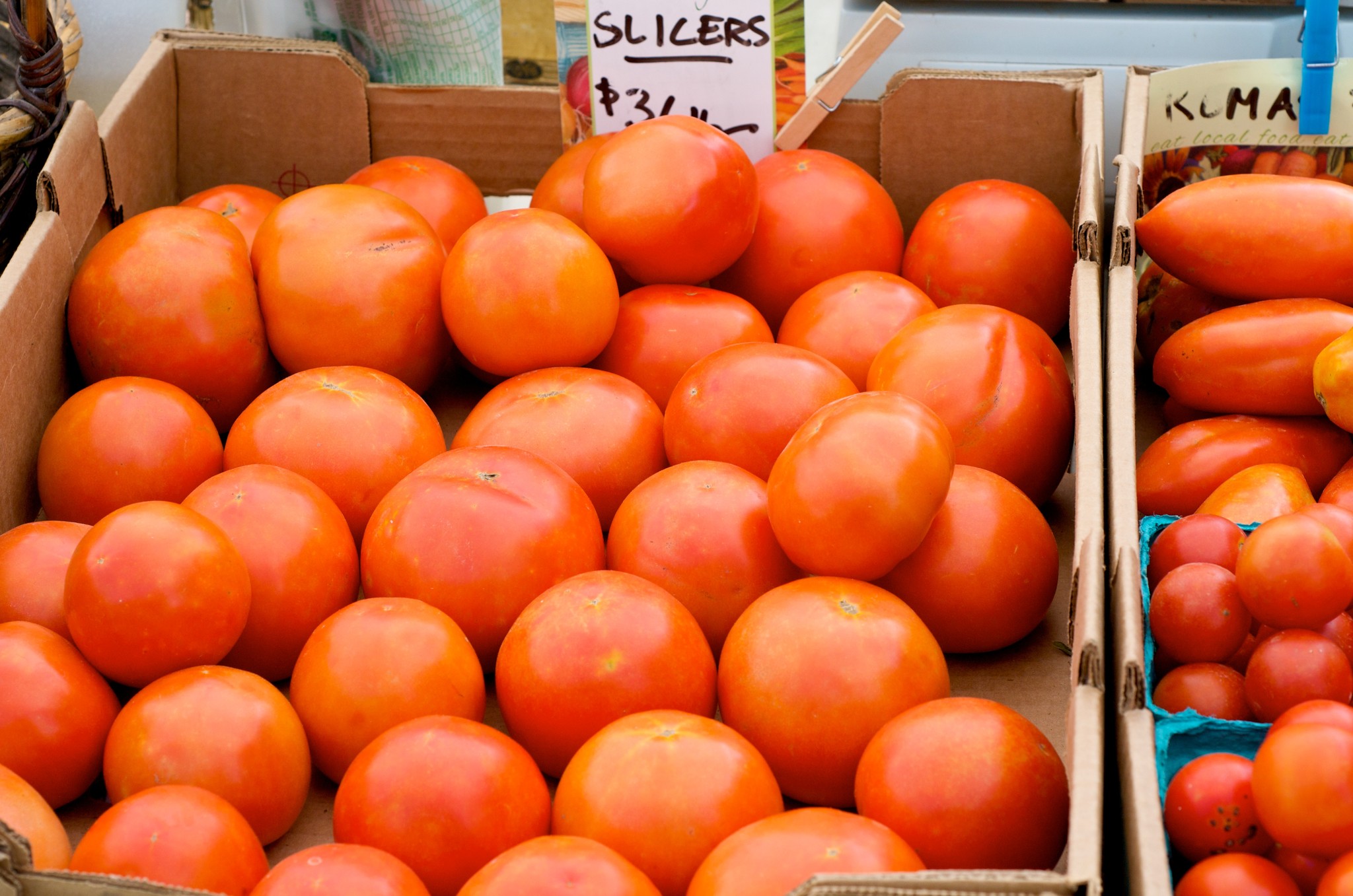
[1219,149,1353,186]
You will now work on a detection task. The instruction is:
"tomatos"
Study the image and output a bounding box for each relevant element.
[0,114,1077,896]
[1136,173,1353,896]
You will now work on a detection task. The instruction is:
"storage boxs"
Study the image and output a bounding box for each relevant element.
[0,28,1353,896]
